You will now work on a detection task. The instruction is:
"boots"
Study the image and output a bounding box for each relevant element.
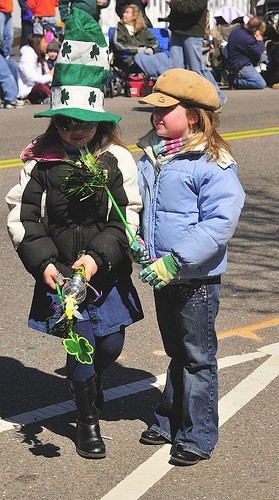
[69,372,106,459]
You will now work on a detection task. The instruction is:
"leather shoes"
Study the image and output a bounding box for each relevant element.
[141,430,170,445]
[171,447,200,465]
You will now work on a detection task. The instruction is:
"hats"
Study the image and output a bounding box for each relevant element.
[48,38,59,52]
[138,68,220,111]
[33,6,122,125]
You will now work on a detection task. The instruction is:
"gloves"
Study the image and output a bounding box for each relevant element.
[130,234,180,291]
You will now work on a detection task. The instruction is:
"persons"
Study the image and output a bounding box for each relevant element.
[130,68,246,466]
[0,0,279,114]
[5,72,144,458]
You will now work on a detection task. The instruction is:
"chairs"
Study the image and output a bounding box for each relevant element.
[108,27,170,96]
